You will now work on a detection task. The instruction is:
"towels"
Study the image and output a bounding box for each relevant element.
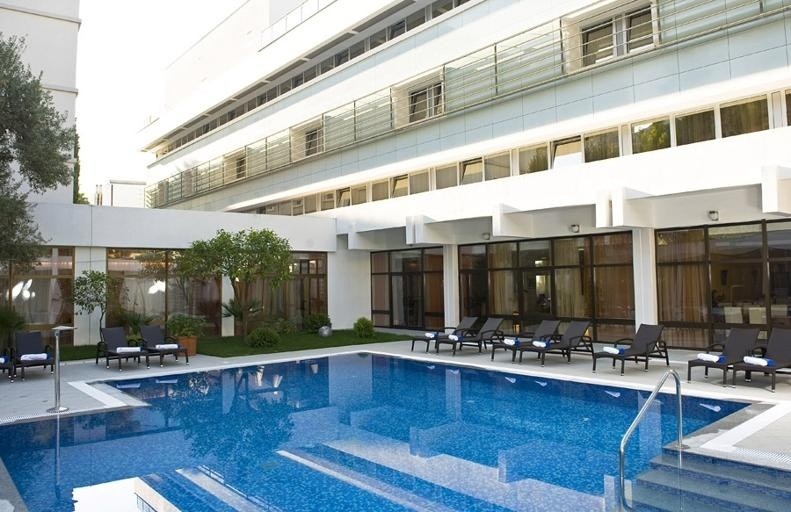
[21,352,49,360]
[156,378,178,383]
[116,346,142,353]
[0,357,5,364]
[155,344,180,350]
[116,382,142,389]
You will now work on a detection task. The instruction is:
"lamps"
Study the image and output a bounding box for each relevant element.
[483,232,490,240]
[708,210,719,221]
[572,225,579,232]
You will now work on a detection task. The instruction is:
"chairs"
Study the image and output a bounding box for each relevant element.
[491,321,560,363]
[593,322,666,376]
[436,318,504,355]
[688,326,760,386]
[0,335,15,384]
[731,327,789,391]
[413,317,477,352]
[10,330,54,382]
[529,321,591,365]
[138,325,191,369]
[95,328,150,372]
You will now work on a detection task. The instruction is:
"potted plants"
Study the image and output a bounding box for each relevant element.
[164,312,207,357]
